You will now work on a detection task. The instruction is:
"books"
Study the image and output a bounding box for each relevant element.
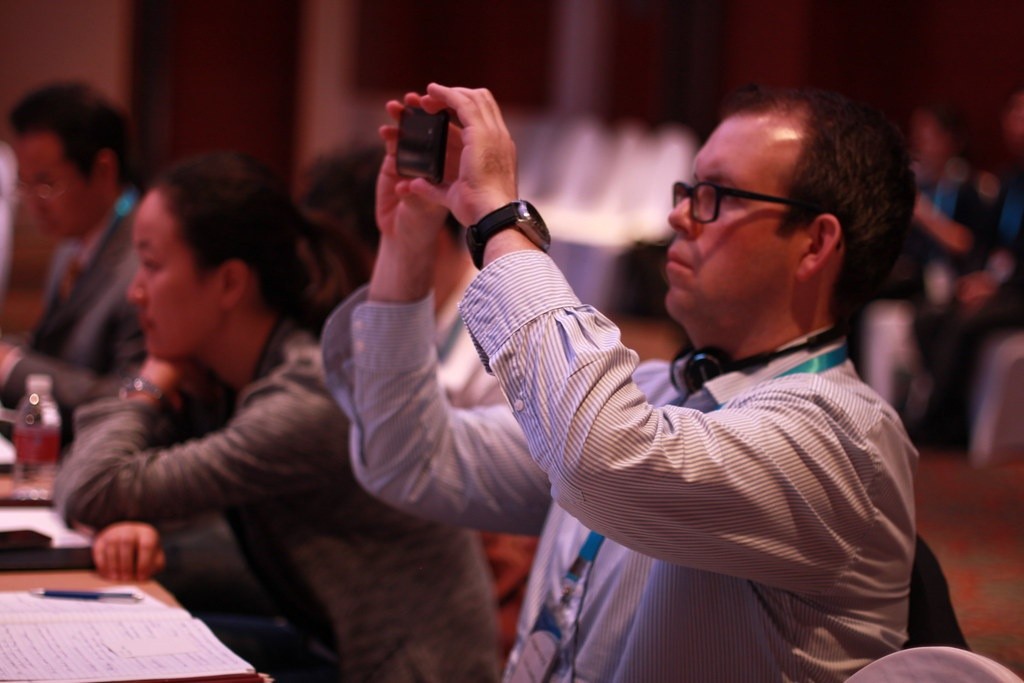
[0,585,255,683]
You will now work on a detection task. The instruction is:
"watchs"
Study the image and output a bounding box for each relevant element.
[466,200,550,270]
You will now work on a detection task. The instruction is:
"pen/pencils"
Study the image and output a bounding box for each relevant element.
[26,586,146,606]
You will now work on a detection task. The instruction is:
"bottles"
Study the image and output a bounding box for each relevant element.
[13,374,61,499]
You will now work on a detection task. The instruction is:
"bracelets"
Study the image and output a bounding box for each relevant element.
[118,377,171,408]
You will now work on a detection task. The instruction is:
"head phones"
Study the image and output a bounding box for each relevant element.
[671,321,847,395]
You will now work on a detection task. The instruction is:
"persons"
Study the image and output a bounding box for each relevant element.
[321,81,919,683]
[0,78,1023,682]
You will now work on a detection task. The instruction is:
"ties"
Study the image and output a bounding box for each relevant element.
[52,253,76,306]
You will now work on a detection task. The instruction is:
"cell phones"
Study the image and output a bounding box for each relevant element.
[396,106,448,183]
[0,529,52,548]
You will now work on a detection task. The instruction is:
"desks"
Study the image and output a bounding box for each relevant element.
[0,475,277,683]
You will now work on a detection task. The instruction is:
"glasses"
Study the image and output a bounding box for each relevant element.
[672,180,796,224]
